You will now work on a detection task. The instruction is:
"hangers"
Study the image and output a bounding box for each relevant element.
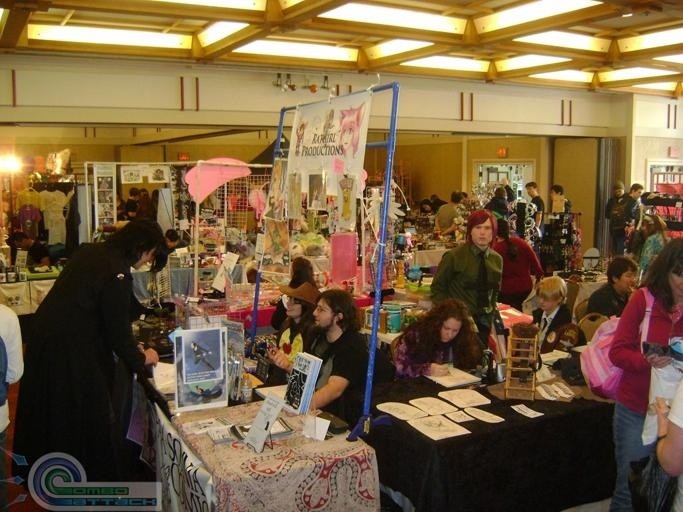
[24,179,37,192]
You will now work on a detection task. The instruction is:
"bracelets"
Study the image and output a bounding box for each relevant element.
[658,433,666,441]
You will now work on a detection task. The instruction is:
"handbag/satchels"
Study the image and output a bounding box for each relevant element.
[641,356,683,447]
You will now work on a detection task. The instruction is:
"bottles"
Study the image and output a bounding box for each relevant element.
[359,306,401,334]
[481,350,493,382]
[241,374,251,403]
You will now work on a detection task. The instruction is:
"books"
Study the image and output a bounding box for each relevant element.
[281,351,323,414]
[265,286,358,426]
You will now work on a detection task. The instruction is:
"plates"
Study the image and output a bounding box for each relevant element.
[583,248,600,268]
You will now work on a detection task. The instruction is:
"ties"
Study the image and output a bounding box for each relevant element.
[476,251,489,309]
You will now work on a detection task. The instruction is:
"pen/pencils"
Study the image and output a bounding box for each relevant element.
[448,371,452,375]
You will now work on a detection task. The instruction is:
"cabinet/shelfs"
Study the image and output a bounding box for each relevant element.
[190,218,226,254]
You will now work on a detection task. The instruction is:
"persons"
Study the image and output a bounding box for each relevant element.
[428,209,504,350]
[587,255,639,322]
[418,179,645,255]
[389,297,481,383]
[527,274,573,356]
[639,214,669,275]
[115,186,158,221]
[9,214,167,493]
[270,256,316,330]
[652,374,683,512]
[491,218,544,314]
[264,280,322,388]
[191,341,217,371]
[606,237,683,511]
[10,231,54,267]
[155,229,178,271]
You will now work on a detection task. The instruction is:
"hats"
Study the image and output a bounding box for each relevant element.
[612,180,624,190]
[279,255,322,305]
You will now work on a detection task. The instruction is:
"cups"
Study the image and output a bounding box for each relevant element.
[0,272,27,283]
[495,363,507,382]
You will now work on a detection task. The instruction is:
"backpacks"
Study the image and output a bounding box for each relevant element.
[608,198,633,232]
[578,286,654,398]
